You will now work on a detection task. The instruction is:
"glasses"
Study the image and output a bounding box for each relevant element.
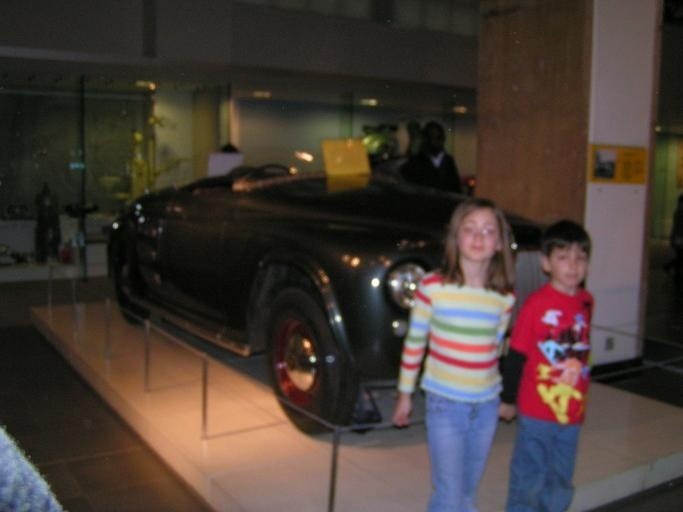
[456,225,501,236]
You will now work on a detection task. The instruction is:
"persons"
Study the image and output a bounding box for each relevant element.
[395,120,462,192]
[390,192,524,511]
[499,218,596,511]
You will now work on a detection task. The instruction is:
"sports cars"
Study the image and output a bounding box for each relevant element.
[99,162,589,436]
